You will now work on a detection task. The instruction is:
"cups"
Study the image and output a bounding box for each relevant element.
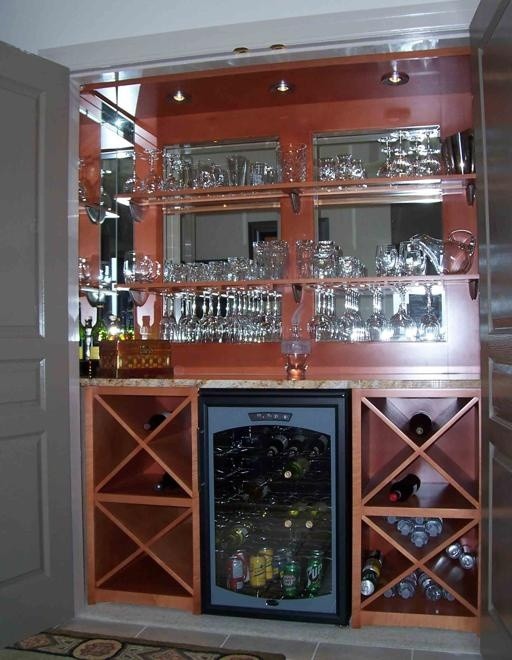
[441,128,474,174]
[319,154,367,192]
[195,155,273,188]
[123,253,161,284]
[78,258,91,287]
[274,142,308,182]
[295,239,427,278]
[162,240,289,282]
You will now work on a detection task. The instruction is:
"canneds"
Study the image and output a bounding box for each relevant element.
[216,546,324,597]
[361,515,475,597]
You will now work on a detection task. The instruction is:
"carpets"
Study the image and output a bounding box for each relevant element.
[0,626,286,660]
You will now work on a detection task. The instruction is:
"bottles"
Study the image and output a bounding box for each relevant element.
[384,573,456,604]
[387,473,421,503]
[153,473,178,492]
[387,516,444,549]
[76,299,135,376]
[220,432,328,548]
[410,410,432,435]
[143,411,172,431]
[279,322,312,379]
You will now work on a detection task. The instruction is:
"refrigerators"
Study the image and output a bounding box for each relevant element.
[197,388,351,625]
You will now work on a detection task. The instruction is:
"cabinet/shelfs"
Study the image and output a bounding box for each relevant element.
[343,386,484,634]
[79,46,483,367]
[81,384,198,611]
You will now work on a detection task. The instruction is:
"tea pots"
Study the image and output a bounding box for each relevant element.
[410,229,477,276]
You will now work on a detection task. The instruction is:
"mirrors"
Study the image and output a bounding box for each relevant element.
[159,126,446,344]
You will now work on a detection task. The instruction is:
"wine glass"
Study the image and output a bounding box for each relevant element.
[158,287,281,344]
[100,169,112,211]
[123,149,185,191]
[78,159,89,202]
[378,129,441,178]
[310,283,447,342]
